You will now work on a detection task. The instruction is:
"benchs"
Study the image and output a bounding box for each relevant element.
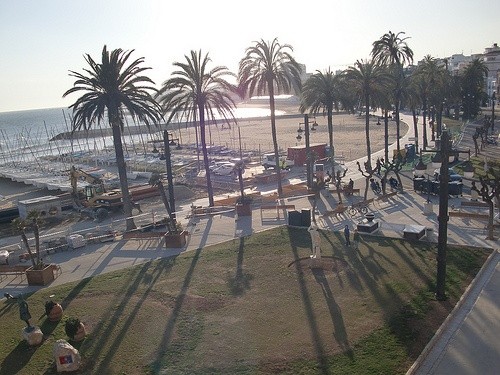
[0,264,63,282]
[328,189,361,196]
[448,212,489,223]
[123,232,166,245]
[318,191,398,221]
[260,204,296,221]
[460,202,490,212]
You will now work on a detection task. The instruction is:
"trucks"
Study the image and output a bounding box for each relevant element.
[262,152,295,171]
[288,143,327,163]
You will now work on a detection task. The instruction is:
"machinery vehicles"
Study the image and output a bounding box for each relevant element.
[70,165,124,220]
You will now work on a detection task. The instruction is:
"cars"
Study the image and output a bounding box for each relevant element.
[208,161,228,174]
[433,167,462,182]
[212,163,238,178]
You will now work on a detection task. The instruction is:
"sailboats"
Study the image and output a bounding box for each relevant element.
[0,108,250,191]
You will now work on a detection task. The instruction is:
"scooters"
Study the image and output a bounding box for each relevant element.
[370,178,382,194]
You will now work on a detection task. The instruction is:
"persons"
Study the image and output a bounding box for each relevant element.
[389,176,395,191]
[307,223,321,257]
[344,225,351,247]
[353,228,361,251]
[18,293,31,332]
[349,178,354,195]
[377,164,381,175]
[337,202,344,215]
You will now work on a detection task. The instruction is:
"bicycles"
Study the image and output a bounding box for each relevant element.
[348,201,368,216]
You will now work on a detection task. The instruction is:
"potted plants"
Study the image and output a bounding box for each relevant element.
[45,300,64,322]
[66,317,86,342]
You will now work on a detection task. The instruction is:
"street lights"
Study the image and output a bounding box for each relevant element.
[414,129,471,299]
[296,114,319,187]
[376,108,396,164]
[146,129,180,224]
